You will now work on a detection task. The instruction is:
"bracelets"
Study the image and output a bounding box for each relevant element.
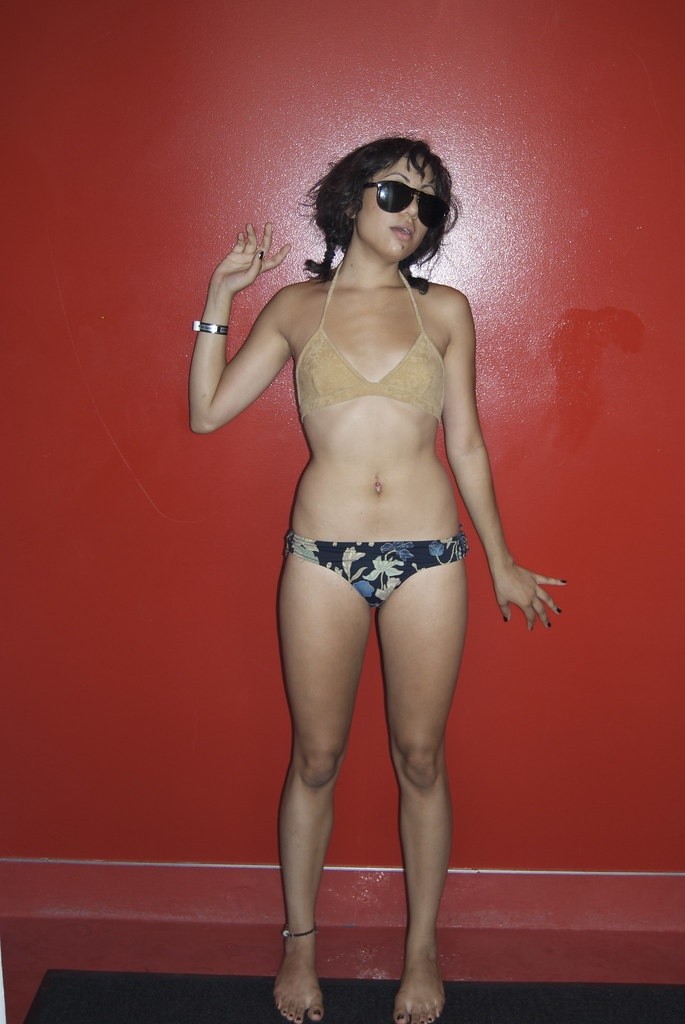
[192,320,228,335]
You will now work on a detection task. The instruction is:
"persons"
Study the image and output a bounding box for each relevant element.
[189,134,573,1024]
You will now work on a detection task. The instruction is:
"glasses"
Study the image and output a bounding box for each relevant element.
[362,180,451,228]
